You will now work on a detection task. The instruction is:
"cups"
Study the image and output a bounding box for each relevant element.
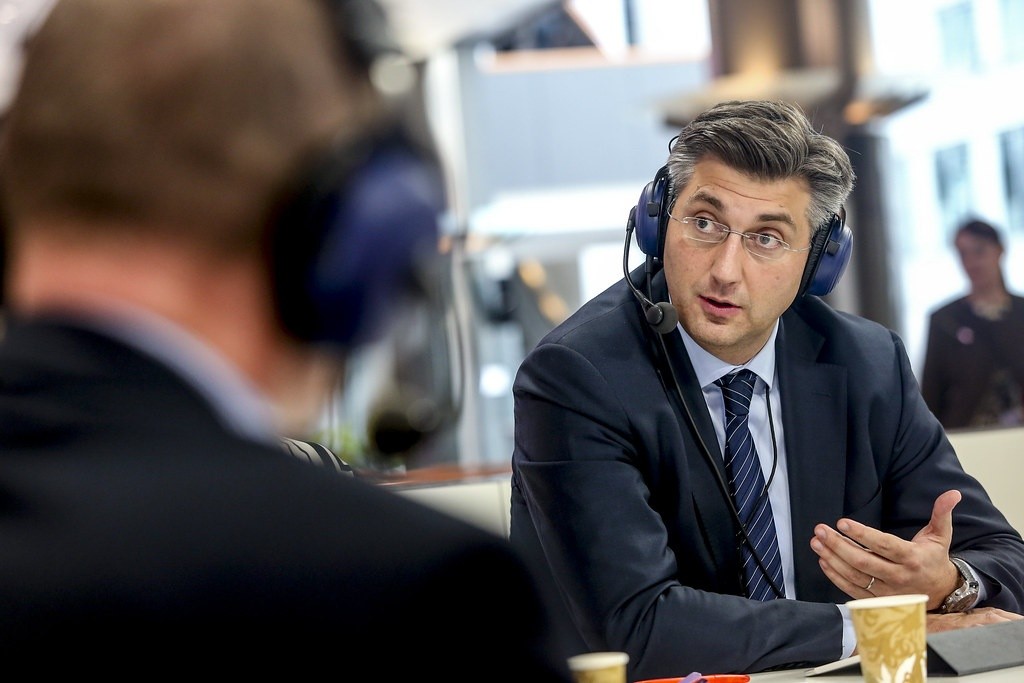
[845,594,930,683]
[567,652,629,683]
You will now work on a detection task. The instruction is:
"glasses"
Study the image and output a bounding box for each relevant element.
[666,197,812,260]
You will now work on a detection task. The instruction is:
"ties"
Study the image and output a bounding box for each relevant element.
[713,370,786,601]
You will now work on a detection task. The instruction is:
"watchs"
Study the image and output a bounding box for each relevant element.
[935,557,979,614]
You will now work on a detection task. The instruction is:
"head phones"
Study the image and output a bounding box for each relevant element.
[626,164,854,300]
[255,0,452,353]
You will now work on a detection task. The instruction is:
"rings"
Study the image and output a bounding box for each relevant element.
[865,577,875,591]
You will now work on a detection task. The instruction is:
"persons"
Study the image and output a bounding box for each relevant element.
[0,0,594,683]
[510,99,1023,683]
[919,220,1024,429]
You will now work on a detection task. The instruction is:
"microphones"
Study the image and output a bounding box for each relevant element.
[367,248,468,459]
[621,207,679,333]
